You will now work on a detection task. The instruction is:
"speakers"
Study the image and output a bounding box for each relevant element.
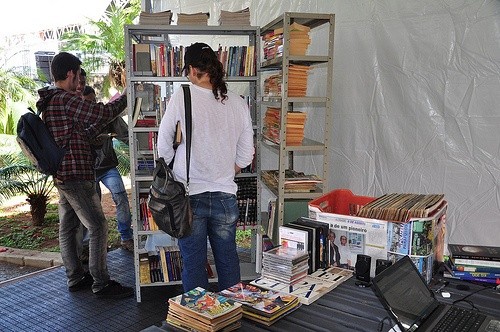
[375,259,392,276]
[355,254,371,286]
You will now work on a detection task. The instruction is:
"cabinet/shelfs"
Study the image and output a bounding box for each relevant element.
[123,23,263,302]
[260,11,336,245]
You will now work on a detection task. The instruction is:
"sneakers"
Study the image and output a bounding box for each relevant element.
[94,280,134,299]
[67,273,93,292]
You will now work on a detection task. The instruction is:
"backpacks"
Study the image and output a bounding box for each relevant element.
[16,106,77,175]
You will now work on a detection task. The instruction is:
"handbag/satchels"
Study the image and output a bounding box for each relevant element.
[147,156,193,238]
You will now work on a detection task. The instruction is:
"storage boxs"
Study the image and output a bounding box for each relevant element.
[307,189,449,283]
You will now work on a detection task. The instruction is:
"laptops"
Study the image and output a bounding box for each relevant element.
[370,254,492,332]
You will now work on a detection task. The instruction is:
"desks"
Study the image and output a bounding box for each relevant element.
[139,274,500,332]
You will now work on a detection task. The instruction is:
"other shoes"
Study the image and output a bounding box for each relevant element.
[121,240,134,251]
[81,255,89,262]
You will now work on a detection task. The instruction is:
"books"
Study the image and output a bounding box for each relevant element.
[139,9,173,25]
[232,178,257,226]
[262,22,311,59]
[218,7,251,26]
[176,12,211,26]
[133,41,255,77]
[443,244,500,284]
[219,281,302,326]
[139,195,159,231]
[133,132,158,170]
[139,245,215,284]
[264,64,311,97]
[133,85,169,128]
[166,287,243,332]
[262,106,307,145]
[261,169,324,193]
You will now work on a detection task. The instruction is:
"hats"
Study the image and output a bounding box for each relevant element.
[180,42,216,73]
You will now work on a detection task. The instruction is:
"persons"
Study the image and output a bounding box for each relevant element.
[38,53,134,297]
[330,231,341,267]
[338,236,351,269]
[157,43,255,293]
